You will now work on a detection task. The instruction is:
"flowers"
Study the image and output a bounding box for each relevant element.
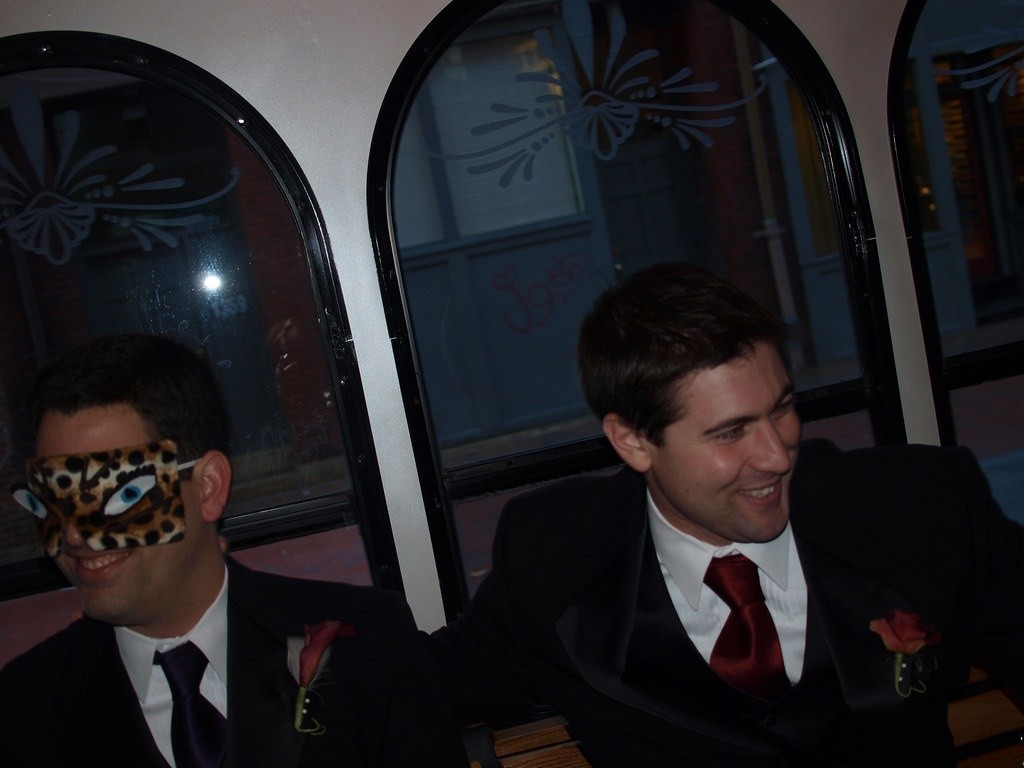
[286,619,356,736]
[868,608,941,697]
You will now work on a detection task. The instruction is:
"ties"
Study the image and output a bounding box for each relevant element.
[153,641,231,768]
[704,553,789,706]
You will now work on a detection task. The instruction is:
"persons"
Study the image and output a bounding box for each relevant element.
[0,335,468,768]
[74,258,1024,768]
